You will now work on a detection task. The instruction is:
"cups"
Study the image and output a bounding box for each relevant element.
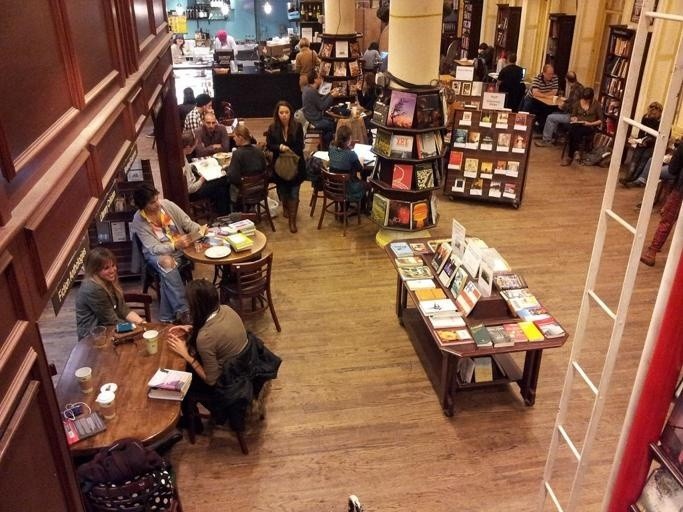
[352,107,357,116]
[141,330,161,355]
[73,367,93,394]
[94,392,117,419]
[88,325,108,347]
[345,102,351,110]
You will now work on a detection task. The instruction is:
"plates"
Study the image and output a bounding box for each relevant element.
[204,246,232,259]
[213,150,232,159]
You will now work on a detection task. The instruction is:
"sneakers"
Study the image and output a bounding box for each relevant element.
[536,139,552,147]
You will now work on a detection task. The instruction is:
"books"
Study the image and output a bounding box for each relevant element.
[64,411,107,448]
[226,233,254,253]
[442,0,472,63]
[632,465,682,512]
[654,386,683,477]
[369,65,531,230]
[388,234,566,384]
[147,367,193,401]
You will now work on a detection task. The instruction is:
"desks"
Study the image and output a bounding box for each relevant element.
[385,235,568,418]
[453,59,601,160]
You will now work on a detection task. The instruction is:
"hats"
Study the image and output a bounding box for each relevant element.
[218,31,227,40]
[195,94,210,107]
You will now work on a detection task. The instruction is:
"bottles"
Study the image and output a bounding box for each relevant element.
[186,4,209,19]
[301,3,322,22]
[210,7,225,19]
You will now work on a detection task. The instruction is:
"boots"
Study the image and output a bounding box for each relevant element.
[283,198,289,217]
[290,200,300,232]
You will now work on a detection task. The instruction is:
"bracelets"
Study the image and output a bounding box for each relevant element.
[189,358,197,366]
[193,361,201,370]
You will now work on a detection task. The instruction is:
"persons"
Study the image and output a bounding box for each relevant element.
[130,180,223,326]
[440,34,682,209]
[639,138,682,266]
[267,37,385,234]
[179,87,266,216]
[171,31,238,64]
[164,278,248,433]
[77,246,147,342]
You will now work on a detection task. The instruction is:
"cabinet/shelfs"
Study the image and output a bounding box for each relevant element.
[462,0,481,59]
[544,14,576,96]
[494,5,520,72]
[442,109,535,209]
[315,32,365,98]
[368,88,447,230]
[598,23,651,150]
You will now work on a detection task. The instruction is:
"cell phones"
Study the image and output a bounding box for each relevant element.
[65,406,82,421]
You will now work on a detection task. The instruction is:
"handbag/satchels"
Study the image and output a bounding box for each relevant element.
[274,153,299,180]
